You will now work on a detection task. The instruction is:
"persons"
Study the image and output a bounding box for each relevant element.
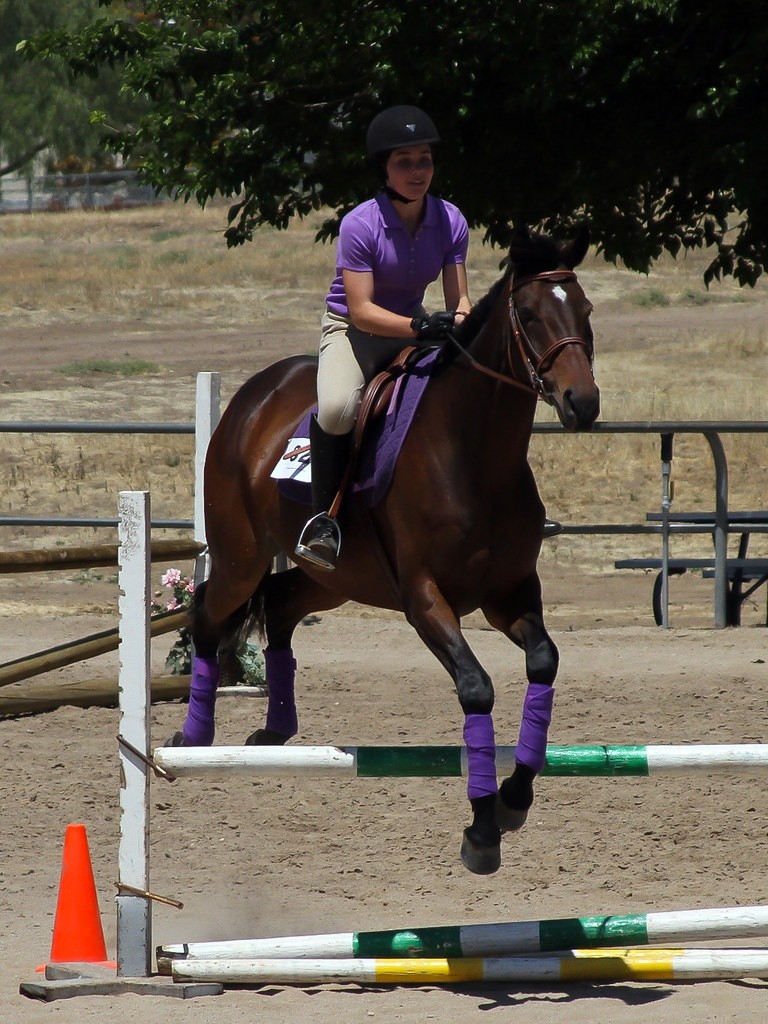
[308,107,473,563]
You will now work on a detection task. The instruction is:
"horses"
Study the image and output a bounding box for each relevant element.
[164,230,607,874]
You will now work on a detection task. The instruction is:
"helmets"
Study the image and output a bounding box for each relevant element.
[365,104,442,157]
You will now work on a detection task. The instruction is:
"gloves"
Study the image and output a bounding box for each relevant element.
[410,310,457,342]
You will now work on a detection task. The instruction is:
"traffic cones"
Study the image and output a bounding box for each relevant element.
[36,825,118,974]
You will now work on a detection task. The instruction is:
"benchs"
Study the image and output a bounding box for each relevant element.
[614,558,768,630]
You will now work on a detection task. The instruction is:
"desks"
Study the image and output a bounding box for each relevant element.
[646,512,768,626]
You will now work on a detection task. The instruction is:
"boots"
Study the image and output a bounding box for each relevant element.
[307,412,357,565]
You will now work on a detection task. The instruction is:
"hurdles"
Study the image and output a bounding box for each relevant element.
[2,367,226,719]
[13,483,768,1008]
